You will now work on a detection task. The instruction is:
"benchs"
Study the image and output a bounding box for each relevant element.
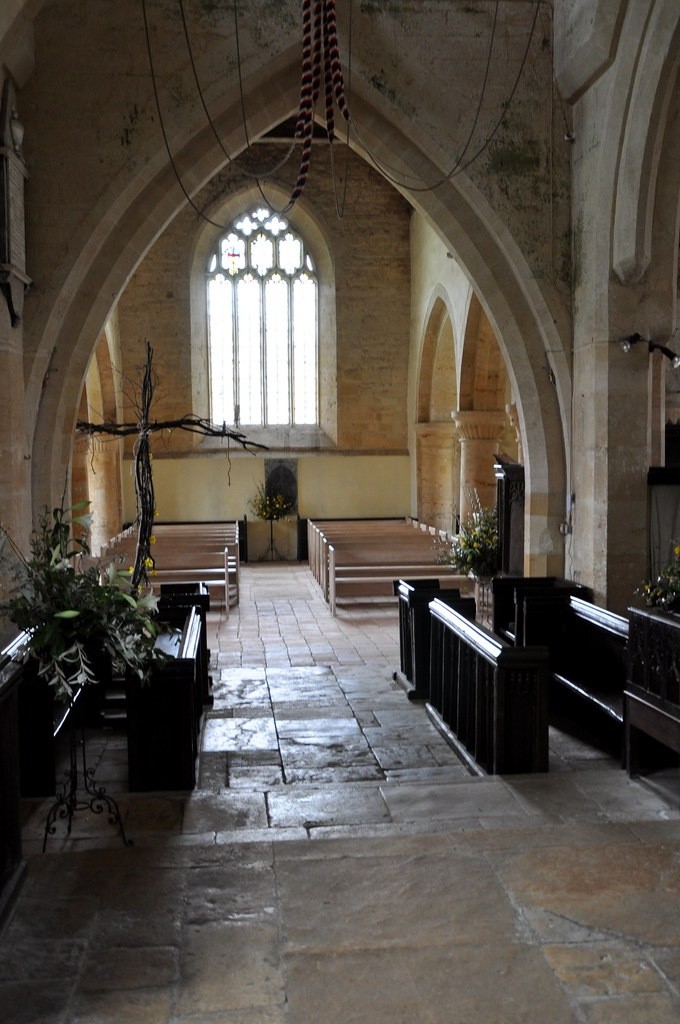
[308,515,476,616]
[126,606,203,793]
[74,520,240,620]
[425,597,549,776]
[551,595,629,723]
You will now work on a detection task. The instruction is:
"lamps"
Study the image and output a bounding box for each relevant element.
[648,339,680,368]
[620,332,641,354]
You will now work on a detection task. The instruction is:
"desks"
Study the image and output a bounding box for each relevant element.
[626,606,680,779]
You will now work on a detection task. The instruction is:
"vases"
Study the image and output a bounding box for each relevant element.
[472,553,497,576]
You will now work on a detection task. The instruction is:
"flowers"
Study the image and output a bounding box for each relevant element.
[431,486,499,576]
[1,466,169,709]
[634,538,680,611]
[247,477,300,524]
[129,452,160,597]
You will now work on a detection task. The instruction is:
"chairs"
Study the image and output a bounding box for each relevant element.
[159,584,214,704]
[390,579,461,698]
[495,577,583,649]
